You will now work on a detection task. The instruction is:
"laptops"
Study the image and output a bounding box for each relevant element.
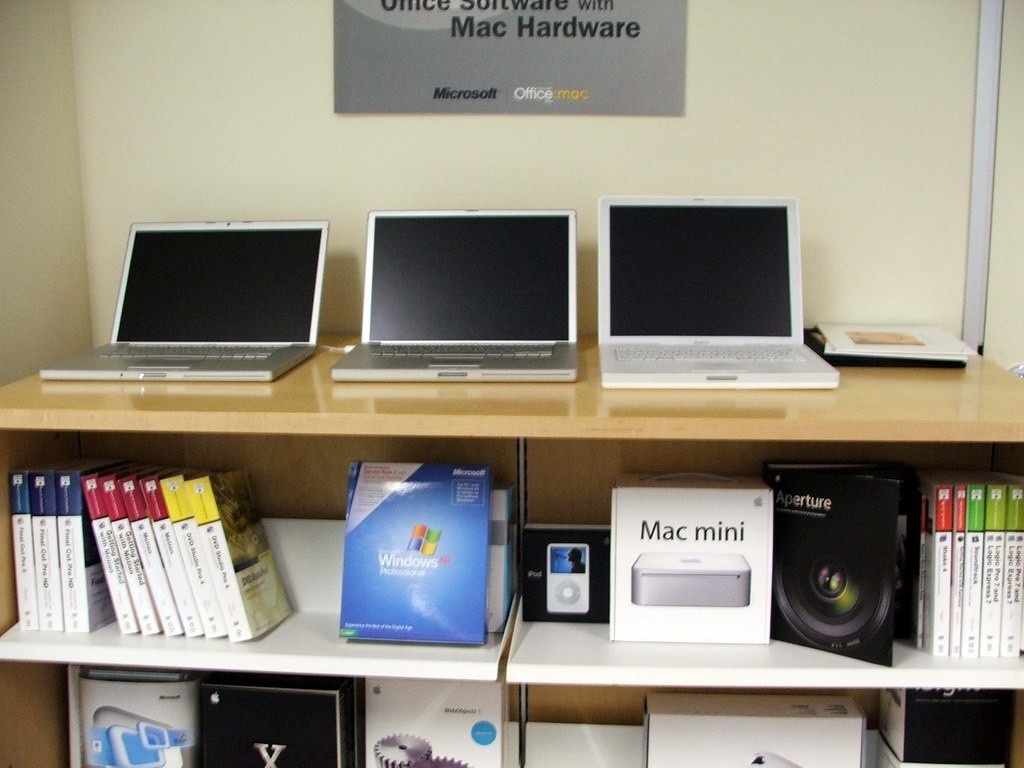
[331,209,578,384]
[597,196,840,390]
[40,219,330,382]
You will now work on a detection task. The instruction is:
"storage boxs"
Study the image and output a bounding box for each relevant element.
[365,657,511,768]
[608,471,774,645]
[877,733,1005,768]
[641,693,868,768]
[877,687,1016,765]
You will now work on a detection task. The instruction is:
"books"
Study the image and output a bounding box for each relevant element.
[804,324,976,369]
[912,470,1024,657]
[8,464,293,643]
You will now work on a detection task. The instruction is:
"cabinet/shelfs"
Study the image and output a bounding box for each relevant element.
[0,336,1024,768]
